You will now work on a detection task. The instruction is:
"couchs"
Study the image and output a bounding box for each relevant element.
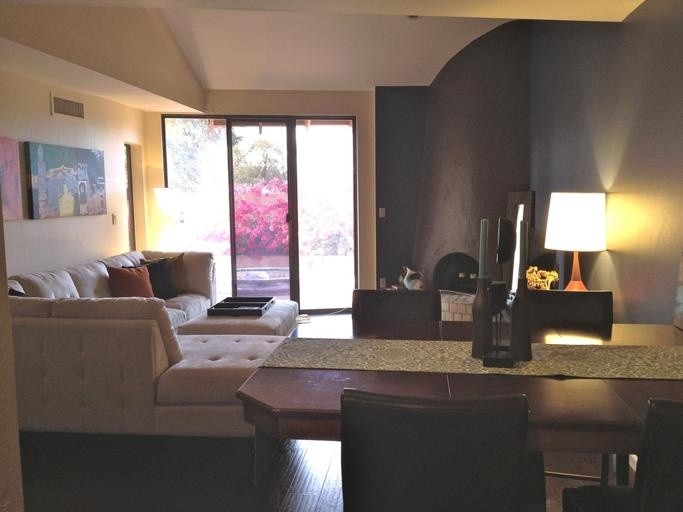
[6,247,290,486]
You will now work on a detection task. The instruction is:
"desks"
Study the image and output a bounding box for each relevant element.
[234,323,683,454]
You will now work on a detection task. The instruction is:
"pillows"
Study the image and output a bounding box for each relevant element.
[103,255,179,300]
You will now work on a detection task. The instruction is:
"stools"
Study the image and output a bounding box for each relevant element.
[562,394,682,510]
[340,385,549,512]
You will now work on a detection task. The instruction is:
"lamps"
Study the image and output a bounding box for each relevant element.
[542,190,609,291]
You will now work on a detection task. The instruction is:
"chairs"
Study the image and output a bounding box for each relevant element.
[350,251,613,344]
